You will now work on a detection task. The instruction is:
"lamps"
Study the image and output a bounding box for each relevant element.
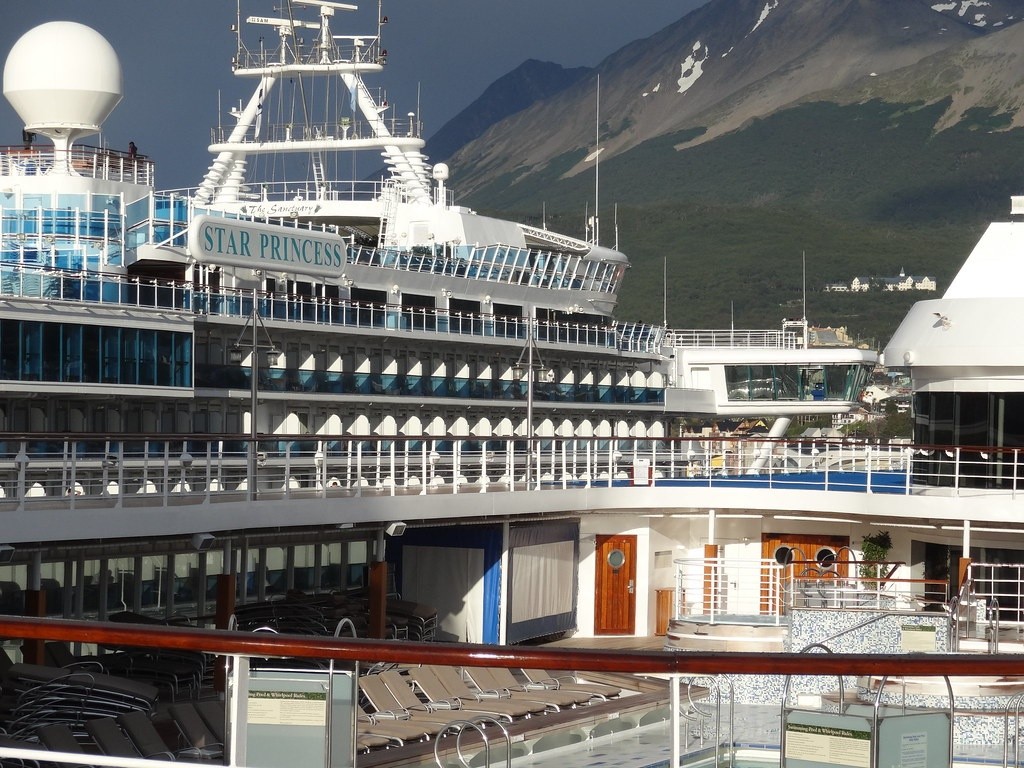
[192,531,215,552]
[385,521,407,536]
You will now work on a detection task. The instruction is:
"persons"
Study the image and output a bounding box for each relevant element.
[128,142,137,158]
[23,125,36,150]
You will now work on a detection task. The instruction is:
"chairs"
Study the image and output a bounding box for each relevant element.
[0,576,622,768]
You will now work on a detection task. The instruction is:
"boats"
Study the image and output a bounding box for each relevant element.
[1,1,916,620]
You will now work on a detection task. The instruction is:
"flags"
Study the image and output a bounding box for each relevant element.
[255,89,263,139]
[349,80,357,112]
